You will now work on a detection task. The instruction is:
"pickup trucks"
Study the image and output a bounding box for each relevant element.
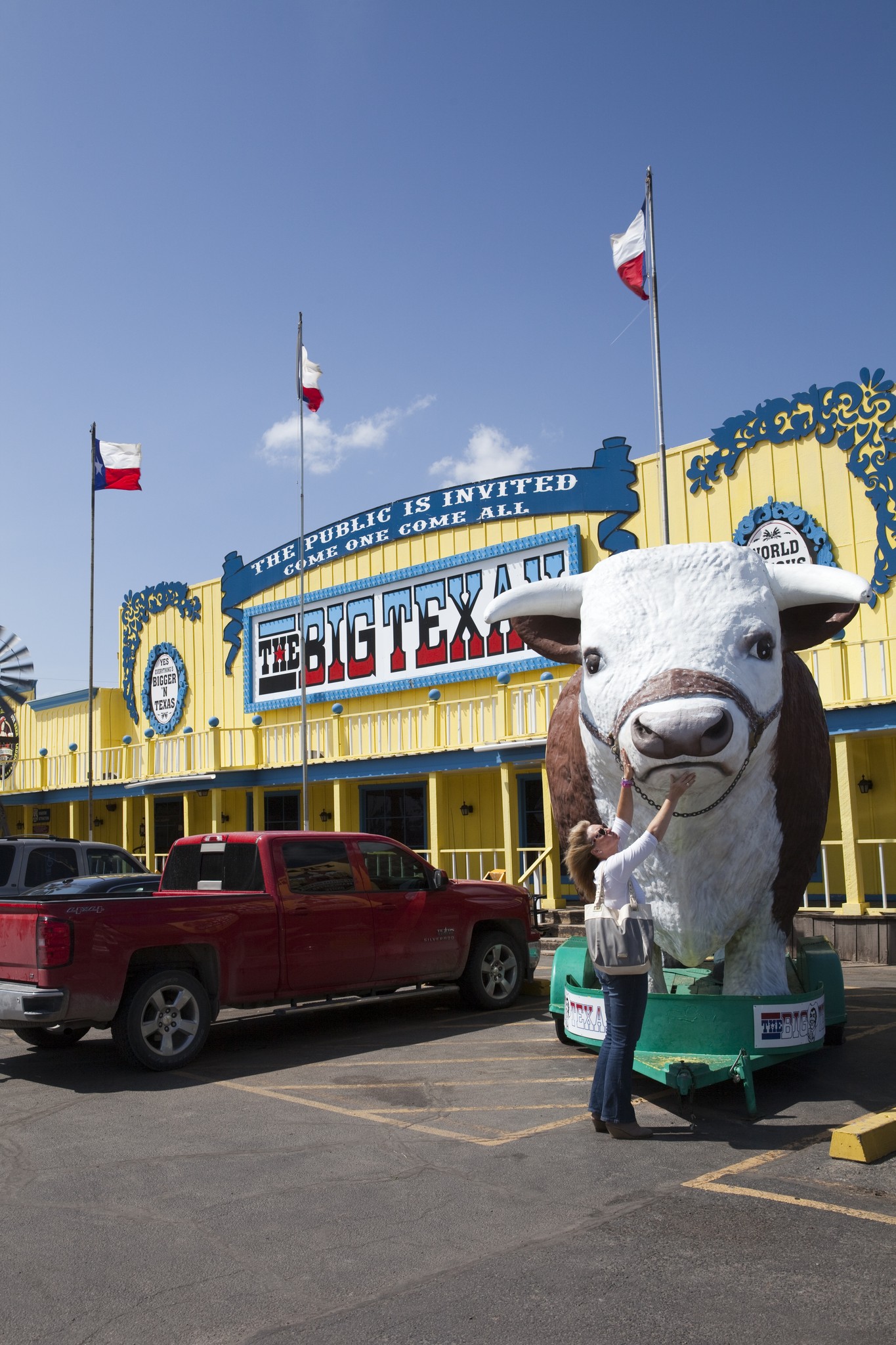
[0,826,545,1072]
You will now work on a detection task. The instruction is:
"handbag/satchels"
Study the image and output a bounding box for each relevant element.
[584,868,654,974]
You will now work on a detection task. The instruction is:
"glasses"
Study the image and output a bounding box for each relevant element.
[591,823,609,847]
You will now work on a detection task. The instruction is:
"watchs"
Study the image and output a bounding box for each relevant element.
[621,775,633,784]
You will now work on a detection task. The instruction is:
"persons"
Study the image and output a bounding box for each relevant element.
[557,742,700,1138]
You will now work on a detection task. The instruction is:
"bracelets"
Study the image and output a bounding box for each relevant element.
[621,782,632,788]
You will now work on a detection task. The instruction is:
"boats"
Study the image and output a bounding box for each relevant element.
[549,935,847,1117]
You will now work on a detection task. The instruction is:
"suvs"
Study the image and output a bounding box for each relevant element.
[0,832,154,894]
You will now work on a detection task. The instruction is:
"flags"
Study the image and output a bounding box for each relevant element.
[91,438,143,491]
[609,186,650,302]
[295,337,325,413]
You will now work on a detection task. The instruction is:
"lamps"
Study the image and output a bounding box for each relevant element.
[221,811,229,823]
[140,823,145,836]
[16,820,24,830]
[93,816,103,827]
[196,789,209,796]
[858,774,873,794]
[319,808,331,821]
[459,801,473,815]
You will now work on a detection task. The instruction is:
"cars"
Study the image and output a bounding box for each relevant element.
[17,873,167,896]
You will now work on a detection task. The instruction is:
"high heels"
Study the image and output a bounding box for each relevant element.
[605,1118,653,1140]
[591,1112,606,1132]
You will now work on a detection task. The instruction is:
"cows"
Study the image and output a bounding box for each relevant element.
[483,543,864,995]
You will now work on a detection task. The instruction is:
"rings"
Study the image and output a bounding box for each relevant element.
[686,782,689,787]
